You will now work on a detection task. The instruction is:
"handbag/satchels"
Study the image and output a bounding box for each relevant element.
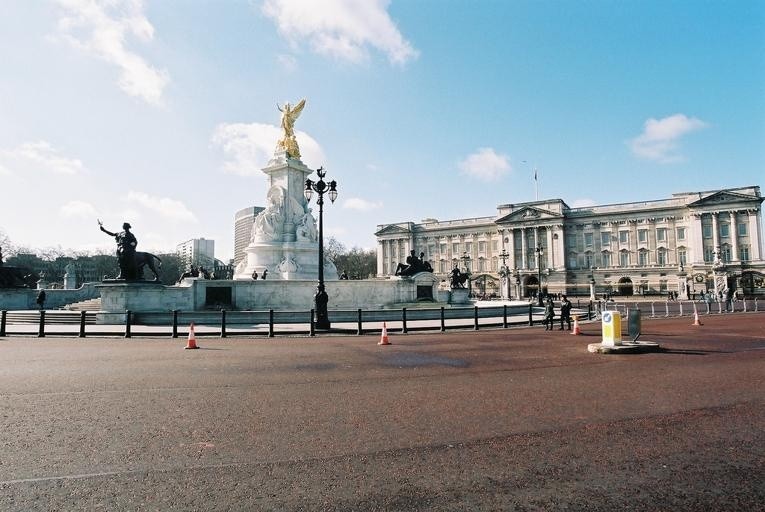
[542,316,549,324]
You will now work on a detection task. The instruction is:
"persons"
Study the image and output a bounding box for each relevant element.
[178,265,194,282]
[717,291,723,313]
[276,102,291,136]
[723,287,732,311]
[338,269,348,279]
[542,299,555,330]
[36,288,45,310]
[100,223,137,279]
[261,269,268,279]
[394,249,433,276]
[699,288,714,314]
[559,295,572,330]
[252,271,258,280]
[449,265,462,289]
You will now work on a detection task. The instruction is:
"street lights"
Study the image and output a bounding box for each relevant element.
[499,249,510,266]
[535,241,544,306]
[304,167,338,328]
[460,250,470,272]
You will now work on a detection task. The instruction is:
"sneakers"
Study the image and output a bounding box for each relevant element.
[545,327,571,331]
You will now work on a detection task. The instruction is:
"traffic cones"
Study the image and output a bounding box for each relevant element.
[184,321,200,348]
[692,307,704,326]
[378,321,391,344]
[570,317,583,335]
